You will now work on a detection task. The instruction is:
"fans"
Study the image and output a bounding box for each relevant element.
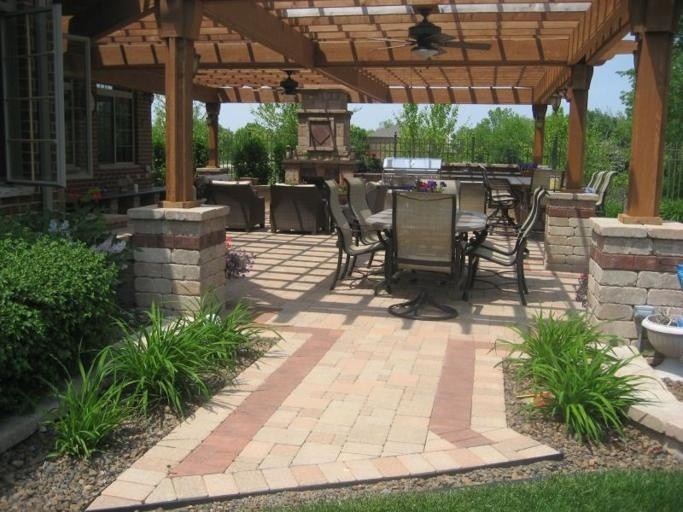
[366,8,490,61]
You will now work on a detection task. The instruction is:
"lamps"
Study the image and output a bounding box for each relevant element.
[409,42,439,60]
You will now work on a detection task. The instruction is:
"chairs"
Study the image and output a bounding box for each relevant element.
[583,167,618,216]
[196,180,328,233]
[319,164,549,310]
